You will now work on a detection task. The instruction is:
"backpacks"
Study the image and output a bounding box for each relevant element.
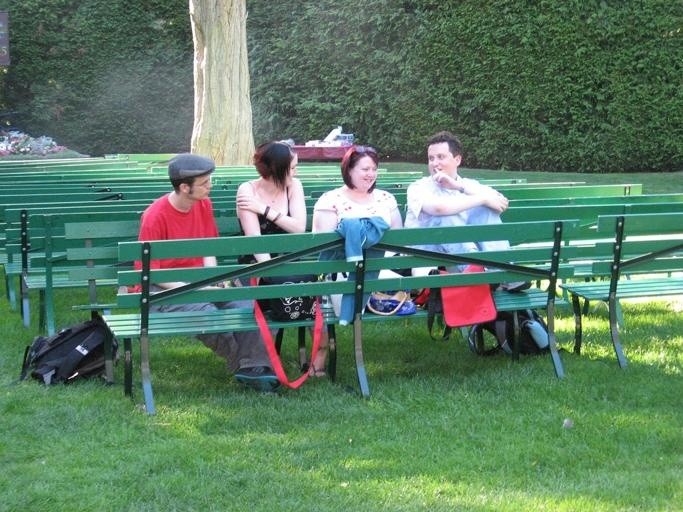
[468,310,549,356]
[26,316,118,384]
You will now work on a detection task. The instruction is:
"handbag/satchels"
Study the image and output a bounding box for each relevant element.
[415,264,497,327]
[253,274,318,321]
[368,290,416,315]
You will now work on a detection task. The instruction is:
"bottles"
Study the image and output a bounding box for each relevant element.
[321,273,335,311]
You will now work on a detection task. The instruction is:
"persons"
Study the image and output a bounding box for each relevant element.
[404,130,533,293]
[310,145,406,248]
[126,153,280,393]
[231,140,330,380]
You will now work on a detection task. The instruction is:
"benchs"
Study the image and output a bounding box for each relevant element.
[0,157,683,416]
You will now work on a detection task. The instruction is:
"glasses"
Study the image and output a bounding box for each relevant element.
[356,146,376,152]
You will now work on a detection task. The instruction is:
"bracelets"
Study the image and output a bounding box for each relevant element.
[262,206,270,218]
[271,213,283,223]
[458,188,464,193]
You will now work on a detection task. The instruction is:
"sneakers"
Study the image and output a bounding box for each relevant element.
[495,280,530,292]
[235,366,282,392]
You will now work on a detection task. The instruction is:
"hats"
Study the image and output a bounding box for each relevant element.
[168,154,216,180]
